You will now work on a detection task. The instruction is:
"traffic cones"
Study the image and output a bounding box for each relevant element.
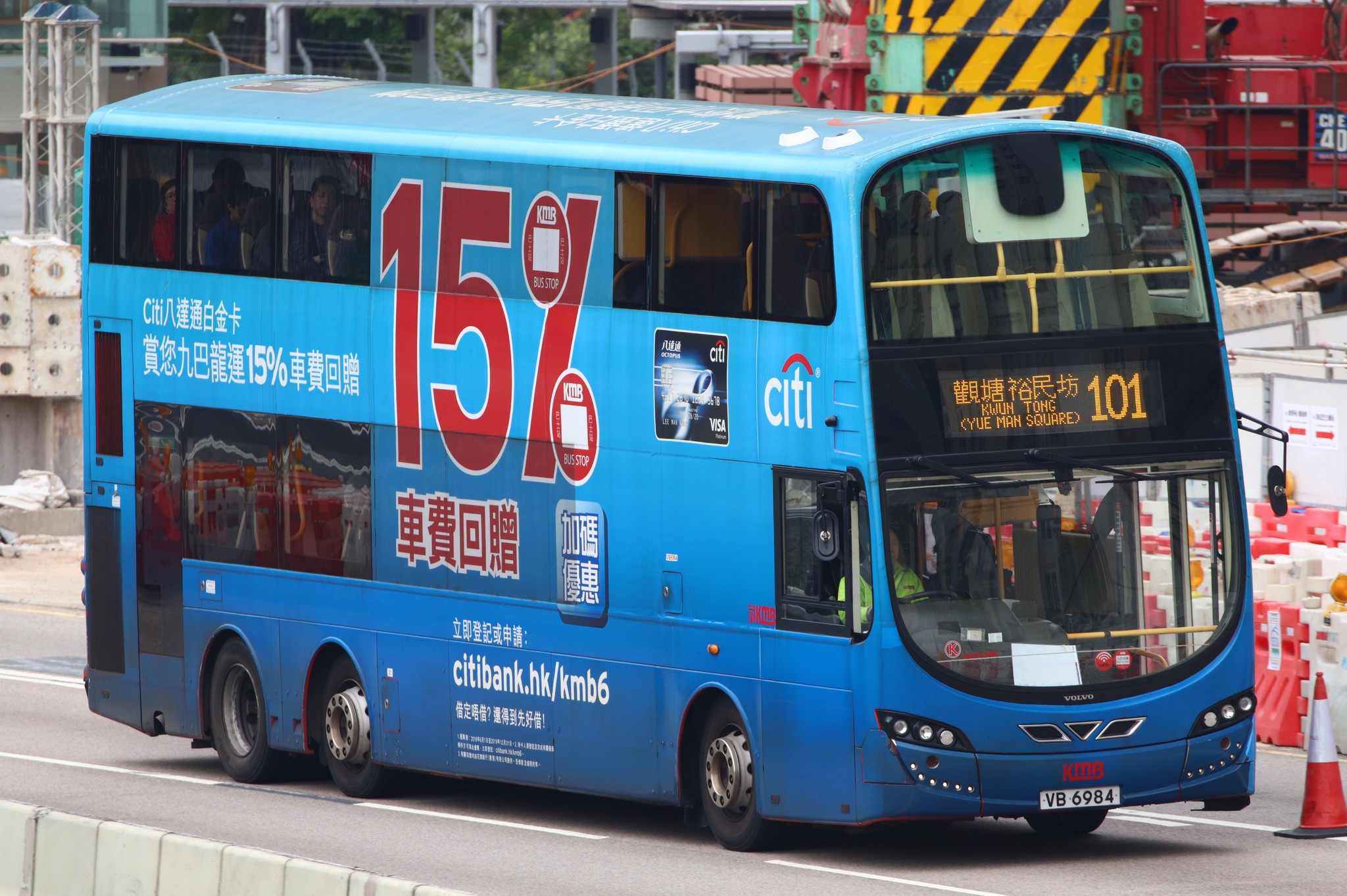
[1273,671,1347,841]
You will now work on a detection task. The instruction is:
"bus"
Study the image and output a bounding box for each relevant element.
[75,69,1297,853]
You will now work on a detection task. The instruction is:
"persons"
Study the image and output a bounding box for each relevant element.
[891,190,932,235]
[837,530,929,633]
[153,178,176,264]
[204,181,254,267]
[291,174,342,277]
[203,156,246,196]
[936,190,963,217]
[931,498,1000,600]
